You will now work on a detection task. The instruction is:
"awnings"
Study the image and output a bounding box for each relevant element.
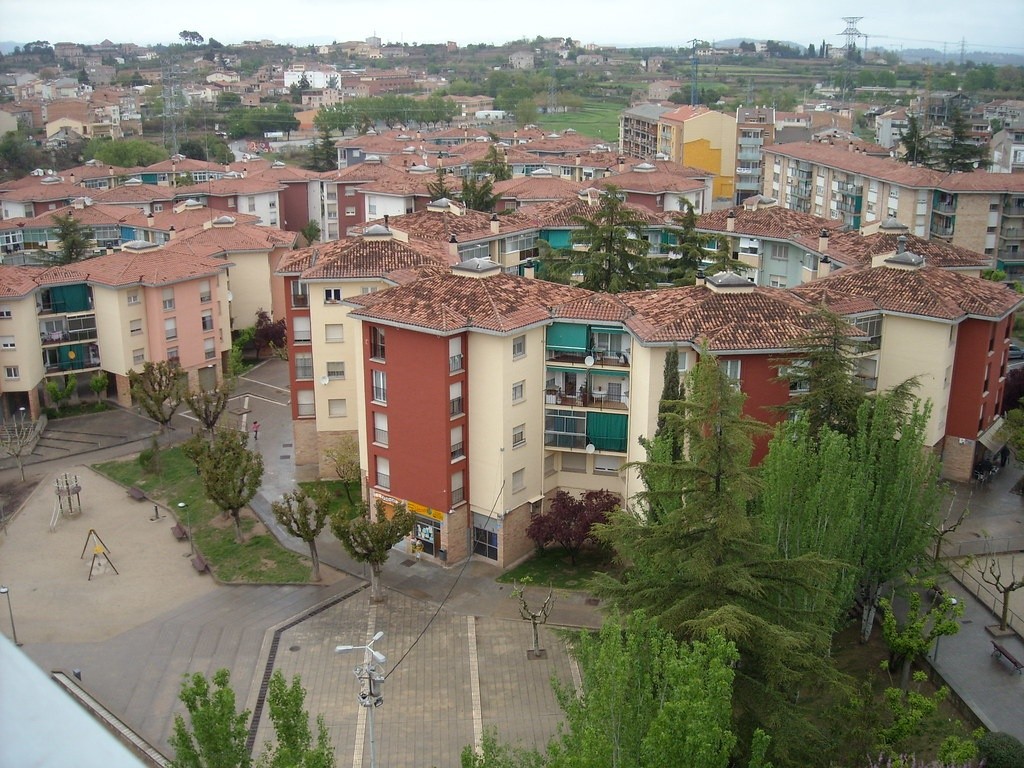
[977,416,1013,455]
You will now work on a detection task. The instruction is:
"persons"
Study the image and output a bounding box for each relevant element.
[975,459,991,472]
[1000,445,1010,467]
[253,421,260,440]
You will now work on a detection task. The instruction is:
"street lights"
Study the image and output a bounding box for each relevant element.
[178,502,194,557]
[20,408,26,432]
[333,631,386,768]
[934,598,957,663]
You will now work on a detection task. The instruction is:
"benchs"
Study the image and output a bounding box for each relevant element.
[171,521,189,542]
[125,486,146,503]
[191,554,208,576]
